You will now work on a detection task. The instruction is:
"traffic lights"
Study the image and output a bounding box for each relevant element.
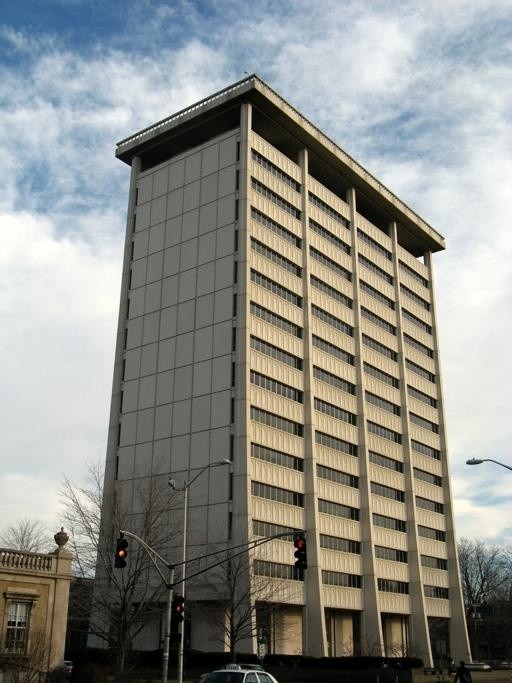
[170,595,185,624]
[114,538,129,570]
[293,536,308,571]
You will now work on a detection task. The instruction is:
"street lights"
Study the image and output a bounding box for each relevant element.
[168,458,232,683]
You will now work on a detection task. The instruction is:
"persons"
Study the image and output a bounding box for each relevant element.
[446,653,453,676]
[453,661,473,683]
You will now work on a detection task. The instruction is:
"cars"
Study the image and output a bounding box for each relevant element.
[198,663,279,683]
